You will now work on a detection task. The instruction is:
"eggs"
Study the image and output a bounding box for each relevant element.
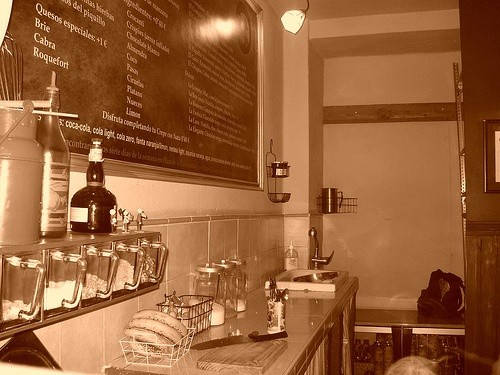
[167,298,212,334]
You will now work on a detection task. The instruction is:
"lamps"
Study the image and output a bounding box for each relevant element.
[280,0,311,35]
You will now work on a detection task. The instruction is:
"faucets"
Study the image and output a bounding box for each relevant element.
[309,227,319,270]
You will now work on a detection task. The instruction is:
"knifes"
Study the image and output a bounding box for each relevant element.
[191,330,289,351]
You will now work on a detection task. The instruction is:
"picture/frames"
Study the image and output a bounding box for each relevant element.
[480,119,500,194]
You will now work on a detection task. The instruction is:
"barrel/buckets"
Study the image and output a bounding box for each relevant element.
[0,100,46,246]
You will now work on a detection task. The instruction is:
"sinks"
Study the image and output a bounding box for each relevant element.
[264,268,349,292]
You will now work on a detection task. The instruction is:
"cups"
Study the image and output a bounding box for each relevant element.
[112,238,146,300]
[138,235,169,291]
[82,242,118,307]
[0,253,46,333]
[267,298,287,334]
[43,245,88,319]
[321,187,343,213]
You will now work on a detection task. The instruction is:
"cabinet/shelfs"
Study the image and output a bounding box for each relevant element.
[355,310,465,375]
[301,285,357,375]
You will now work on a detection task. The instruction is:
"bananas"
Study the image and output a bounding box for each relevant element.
[123,309,187,358]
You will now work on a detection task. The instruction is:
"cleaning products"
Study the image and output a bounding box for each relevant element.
[283,241,299,270]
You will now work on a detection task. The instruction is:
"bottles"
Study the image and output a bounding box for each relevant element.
[229,258,248,313]
[194,262,227,326]
[409,335,464,375]
[283,241,299,272]
[37,86,72,240]
[213,259,238,320]
[70,138,118,234]
[353,337,393,375]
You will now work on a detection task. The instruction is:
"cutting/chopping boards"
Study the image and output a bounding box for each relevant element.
[196,339,288,375]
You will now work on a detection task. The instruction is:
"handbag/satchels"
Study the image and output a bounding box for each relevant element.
[418,269,464,316]
